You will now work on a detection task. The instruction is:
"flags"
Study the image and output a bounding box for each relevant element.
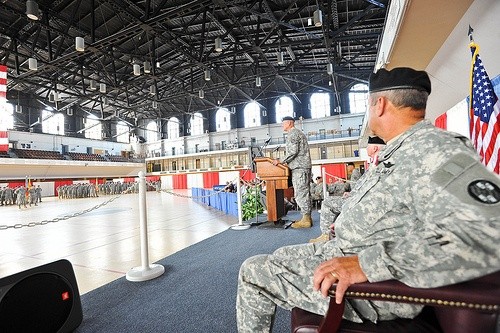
[467,35,500,176]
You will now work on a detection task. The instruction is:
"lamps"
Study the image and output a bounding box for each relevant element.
[75,12,84,51]
[104,98,120,117]
[16,83,22,113]
[133,36,160,108]
[90,70,106,93]
[199,89,204,99]
[277,38,283,64]
[215,36,222,52]
[29,58,37,70]
[49,73,73,116]
[26,0,39,20]
[256,77,261,87]
[204,71,211,81]
[327,64,333,75]
[314,10,322,26]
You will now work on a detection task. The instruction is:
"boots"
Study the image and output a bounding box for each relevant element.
[291,214,313,229]
[309,232,330,243]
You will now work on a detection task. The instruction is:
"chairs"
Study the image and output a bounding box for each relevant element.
[292,271,500,333]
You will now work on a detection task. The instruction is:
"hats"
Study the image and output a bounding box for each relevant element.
[282,116,295,122]
[369,67,431,96]
[316,177,322,180]
[346,162,354,166]
[368,136,386,145]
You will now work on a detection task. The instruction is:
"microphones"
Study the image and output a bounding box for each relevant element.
[271,145,281,153]
[265,137,271,145]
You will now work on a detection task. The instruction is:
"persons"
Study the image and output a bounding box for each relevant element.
[309,176,324,206]
[309,136,388,244]
[102,181,139,195]
[146,179,161,192]
[236,66,500,333]
[105,155,126,162]
[0,184,43,209]
[327,177,351,196]
[347,161,361,189]
[274,116,313,228]
[56,182,100,199]
[11,149,64,160]
[225,179,266,193]
[66,152,107,161]
